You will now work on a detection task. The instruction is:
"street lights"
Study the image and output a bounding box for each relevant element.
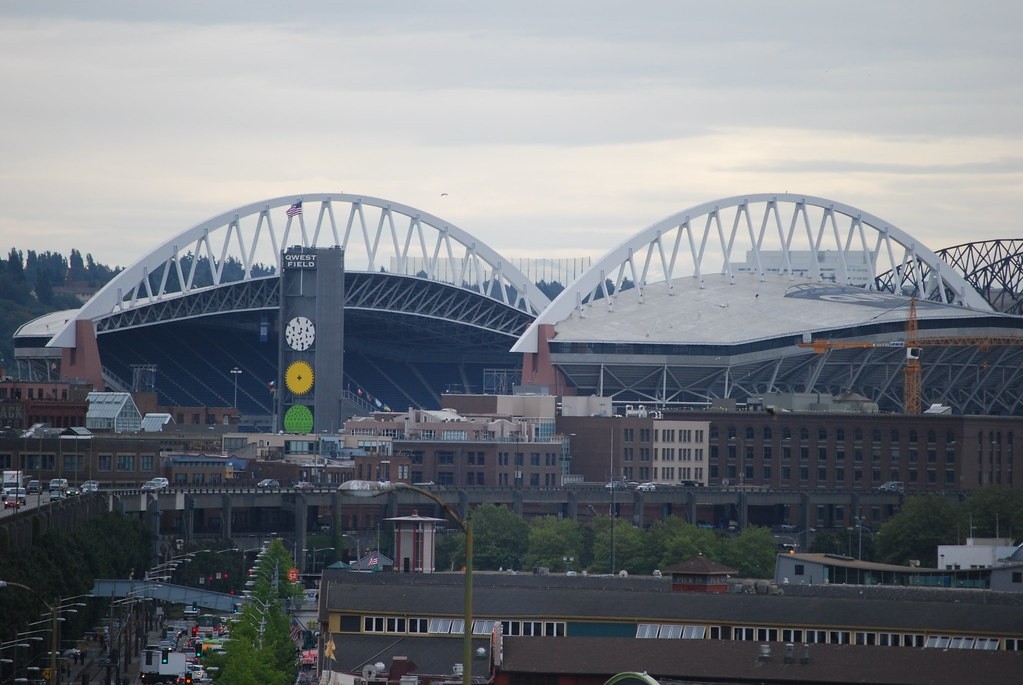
[314,430,329,482]
[342,534,360,563]
[0,539,281,685]
[276,537,296,570]
[312,545,335,575]
[231,366,242,407]
[855,516,866,585]
[336,480,472,685]
[0,425,100,515]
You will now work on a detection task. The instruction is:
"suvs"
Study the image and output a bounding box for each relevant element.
[151,477,169,492]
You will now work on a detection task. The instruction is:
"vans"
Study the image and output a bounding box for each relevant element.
[879,481,906,495]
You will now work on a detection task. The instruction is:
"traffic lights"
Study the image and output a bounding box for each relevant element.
[234,606,238,614]
[162,648,168,664]
[185,673,192,685]
[195,644,202,658]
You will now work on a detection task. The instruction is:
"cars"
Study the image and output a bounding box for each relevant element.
[605,479,706,493]
[293,481,315,491]
[259,478,280,489]
[697,519,863,556]
[142,481,162,493]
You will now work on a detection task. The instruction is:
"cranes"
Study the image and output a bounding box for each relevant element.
[796,294,1023,416]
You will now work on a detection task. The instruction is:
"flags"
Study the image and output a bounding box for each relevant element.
[286,201,303,220]
[268,379,276,386]
[290,617,304,639]
[358,389,391,412]
[368,556,378,566]
